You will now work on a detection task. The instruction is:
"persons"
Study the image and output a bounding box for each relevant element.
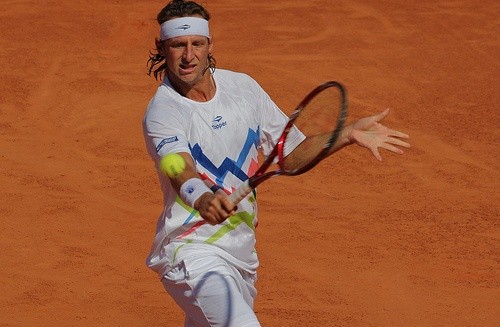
[142,1,411,327]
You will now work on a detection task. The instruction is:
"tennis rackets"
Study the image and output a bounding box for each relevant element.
[219,81,347,223]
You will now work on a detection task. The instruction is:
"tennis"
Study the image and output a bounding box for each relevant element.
[161,154,185,177]
